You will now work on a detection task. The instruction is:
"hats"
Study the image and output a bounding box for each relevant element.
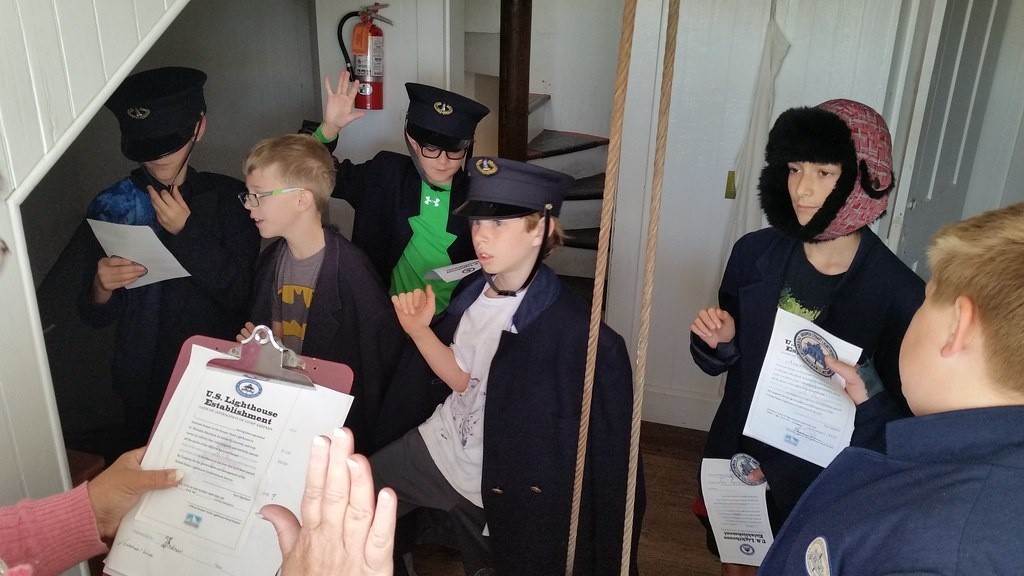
[758,99,896,243]
[404,82,491,152]
[104,67,208,164]
[452,157,576,220]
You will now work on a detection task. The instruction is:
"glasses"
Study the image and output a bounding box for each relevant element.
[238,188,309,208]
[418,141,468,161]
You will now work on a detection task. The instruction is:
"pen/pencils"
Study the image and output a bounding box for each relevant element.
[168,185,175,197]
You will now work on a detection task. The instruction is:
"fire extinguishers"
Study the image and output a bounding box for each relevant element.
[337,2,393,109]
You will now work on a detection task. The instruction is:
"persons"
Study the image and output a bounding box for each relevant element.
[76,65,261,440]
[689,97,927,576]
[369,155,647,576]
[0,426,398,576]
[237,135,396,430]
[759,204,1024,575]
[297,70,491,318]
